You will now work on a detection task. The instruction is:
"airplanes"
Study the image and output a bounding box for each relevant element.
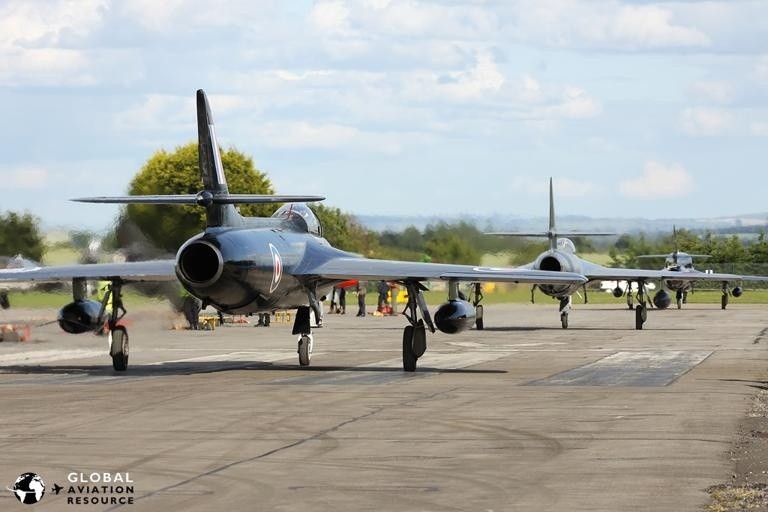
[635,224,767,310]
[0,90,589,371]
[485,177,759,328]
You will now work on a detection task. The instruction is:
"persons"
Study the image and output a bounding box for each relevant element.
[253,312,271,328]
[386,281,401,318]
[356,282,367,318]
[308,287,323,329]
[339,288,346,315]
[327,287,342,315]
[376,280,390,313]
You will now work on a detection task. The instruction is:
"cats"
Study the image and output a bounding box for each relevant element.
[0,90,589,371]
